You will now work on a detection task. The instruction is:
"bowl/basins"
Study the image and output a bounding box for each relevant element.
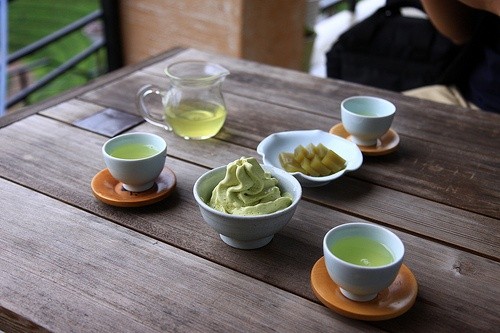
[101,131,167,192]
[256,129,364,187]
[340,95,397,147]
[193,164,302,250]
[323,221,406,303]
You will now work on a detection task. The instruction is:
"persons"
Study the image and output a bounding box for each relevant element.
[401,0,500,114]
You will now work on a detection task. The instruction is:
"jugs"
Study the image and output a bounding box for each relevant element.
[135,60,232,141]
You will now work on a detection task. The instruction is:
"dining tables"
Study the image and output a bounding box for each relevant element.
[0,44,500,333]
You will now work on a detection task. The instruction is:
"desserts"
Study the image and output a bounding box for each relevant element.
[210,156,293,216]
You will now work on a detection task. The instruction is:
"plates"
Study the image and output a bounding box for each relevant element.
[91,165,177,208]
[310,255,419,321]
[331,123,400,155]
